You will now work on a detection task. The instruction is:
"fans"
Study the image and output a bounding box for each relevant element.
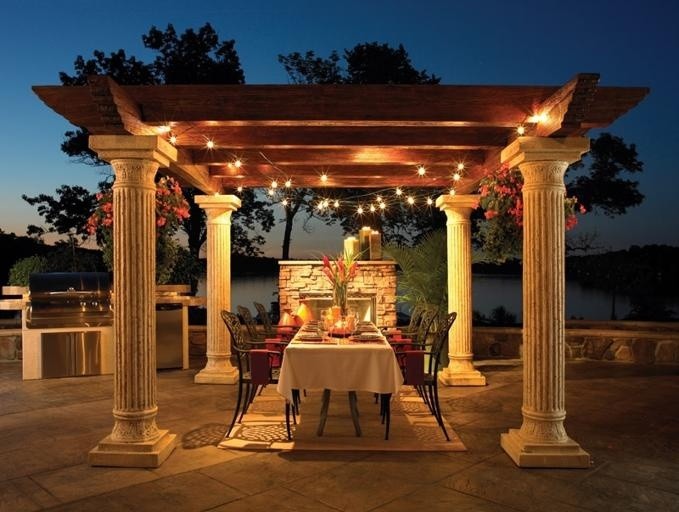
[302,242,370,315]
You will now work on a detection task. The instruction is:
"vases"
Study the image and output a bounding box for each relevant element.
[330,282,348,320]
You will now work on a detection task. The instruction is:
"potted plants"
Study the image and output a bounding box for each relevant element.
[377,227,485,375]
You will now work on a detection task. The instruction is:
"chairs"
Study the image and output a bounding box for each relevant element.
[217,302,306,440]
[374,301,462,442]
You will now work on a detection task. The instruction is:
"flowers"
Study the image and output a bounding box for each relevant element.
[471,163,587,232]
[86,175,191,291]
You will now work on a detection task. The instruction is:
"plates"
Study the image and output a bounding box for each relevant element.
[350,320,386,341]
[300,319,329,340]
